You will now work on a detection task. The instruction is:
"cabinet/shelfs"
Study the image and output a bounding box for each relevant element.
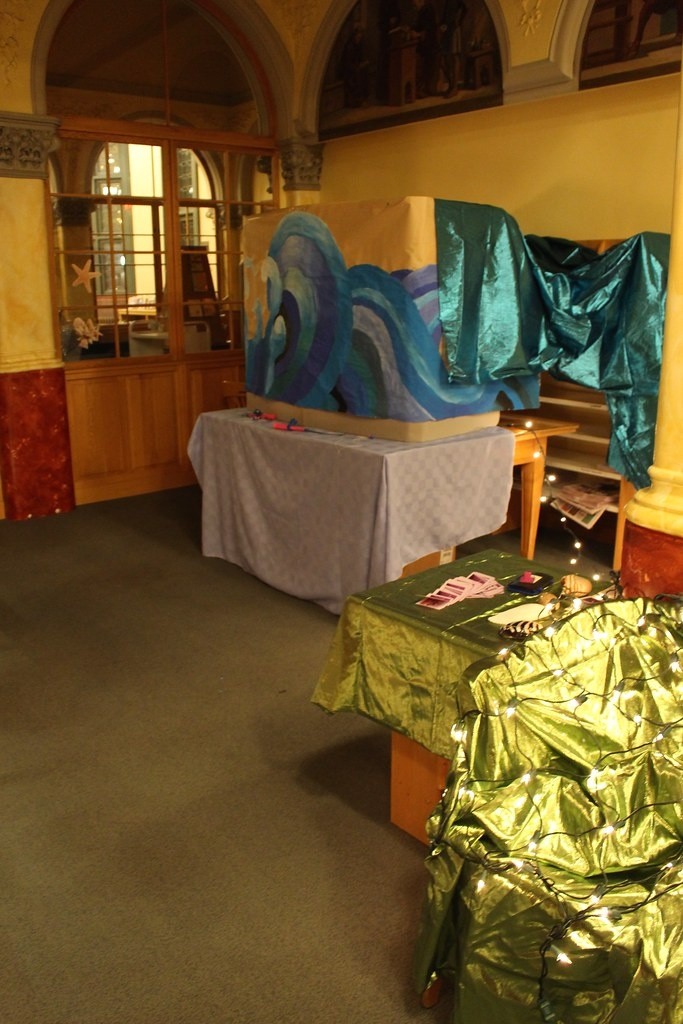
[452,232,674,573]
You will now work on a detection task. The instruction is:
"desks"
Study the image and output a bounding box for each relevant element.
[184,411,580,616]
[309,549,625,848]
